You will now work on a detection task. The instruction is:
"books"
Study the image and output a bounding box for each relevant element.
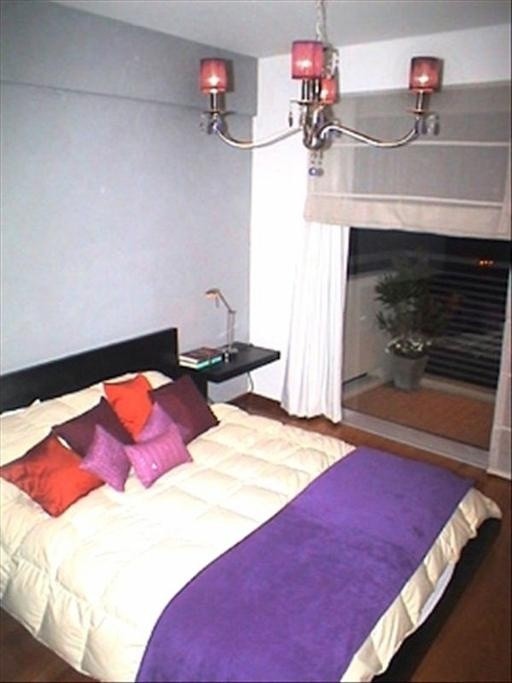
[178,347,223,370]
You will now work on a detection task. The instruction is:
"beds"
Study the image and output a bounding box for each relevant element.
[0,326,504,681]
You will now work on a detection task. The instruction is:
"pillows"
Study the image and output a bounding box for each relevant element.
[0,368,221,517]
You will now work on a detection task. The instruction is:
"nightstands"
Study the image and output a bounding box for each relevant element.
[180,340,281,401]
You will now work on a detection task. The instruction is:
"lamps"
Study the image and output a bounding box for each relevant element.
[204,286,240,354]
[198,0,445,179]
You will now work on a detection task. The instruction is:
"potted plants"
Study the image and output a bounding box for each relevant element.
[373,244,439,390]
